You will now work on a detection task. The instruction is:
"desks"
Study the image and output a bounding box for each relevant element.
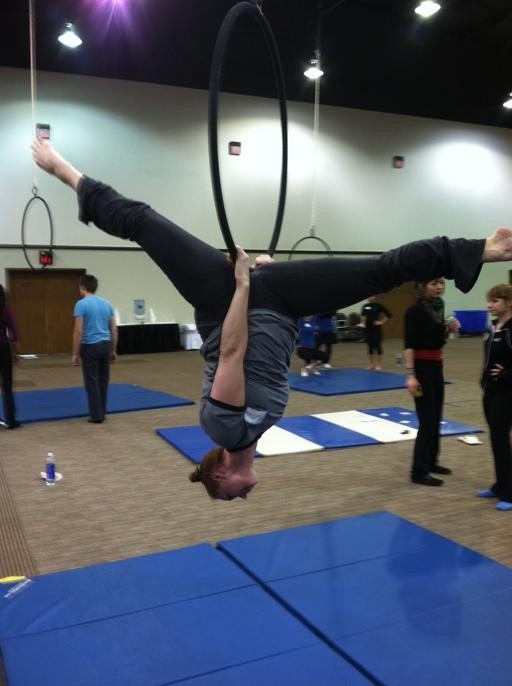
[182,324,203,351]
[116,322,181,355]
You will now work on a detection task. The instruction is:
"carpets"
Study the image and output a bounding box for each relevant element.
[0,510,512,686]
[155,406,486,464]
[288,367,455,396]
[0,383,195,427]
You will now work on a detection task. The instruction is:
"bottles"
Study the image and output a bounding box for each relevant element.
[396,353,402,367]
[45,451,57,487]
[448,312,458,340]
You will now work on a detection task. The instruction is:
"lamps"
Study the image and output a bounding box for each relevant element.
[55,22,87,50]
[414,1,441,17]
[303,67,324,79]
[502,91,512,110]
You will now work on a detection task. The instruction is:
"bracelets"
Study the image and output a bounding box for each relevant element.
[405,367,417,376]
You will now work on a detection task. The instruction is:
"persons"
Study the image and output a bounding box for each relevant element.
[314,310,337,369]
[31,133,512,500]
[360,294,393,371]
[472,283,512,512]
[0,285,21,431]
[70,275,118,422]
[401,277,462,488]
[295,316,329,378]
